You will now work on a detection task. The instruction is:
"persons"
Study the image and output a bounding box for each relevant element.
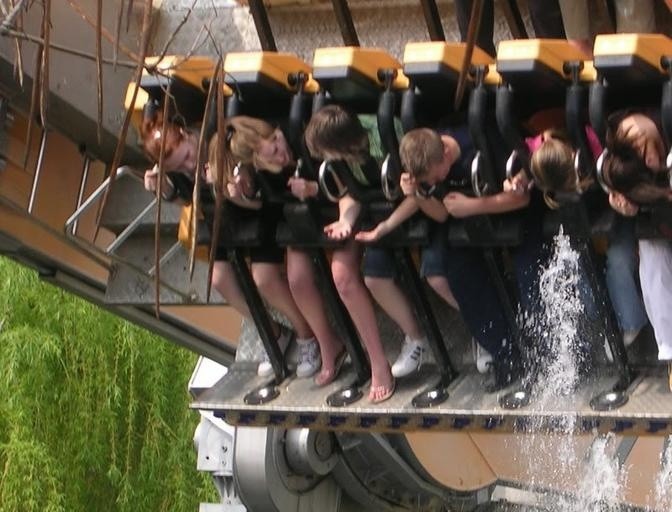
[207,116,397,401]
[399,126,561,393]
[306,106,494,376]
[143,111,321,383]
[605,112,671,393]
[502,127,646,364]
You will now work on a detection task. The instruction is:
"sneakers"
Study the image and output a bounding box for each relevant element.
[257,323,430,404]
[473,336,521,392]
